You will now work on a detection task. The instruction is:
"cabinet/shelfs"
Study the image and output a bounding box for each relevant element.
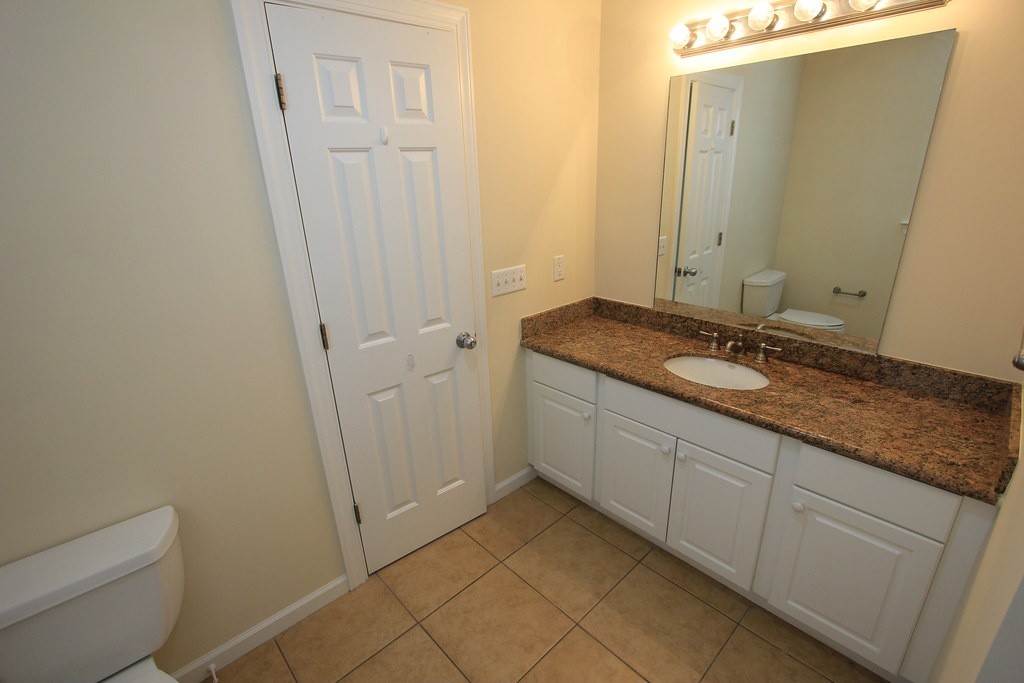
[522,351,1024,683]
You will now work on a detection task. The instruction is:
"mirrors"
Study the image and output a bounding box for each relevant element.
[650,29,959,351]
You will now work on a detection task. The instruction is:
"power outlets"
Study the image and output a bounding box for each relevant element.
[553,255,566,281]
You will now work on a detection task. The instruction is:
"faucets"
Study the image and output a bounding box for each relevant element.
[756,324,766,330]
[725,334,747,356]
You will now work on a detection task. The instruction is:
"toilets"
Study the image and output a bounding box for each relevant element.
[742,269,845,333]
[0,503,187,683]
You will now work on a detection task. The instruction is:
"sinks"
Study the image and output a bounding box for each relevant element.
[662,352,771,391]
[737,323,817,341]
